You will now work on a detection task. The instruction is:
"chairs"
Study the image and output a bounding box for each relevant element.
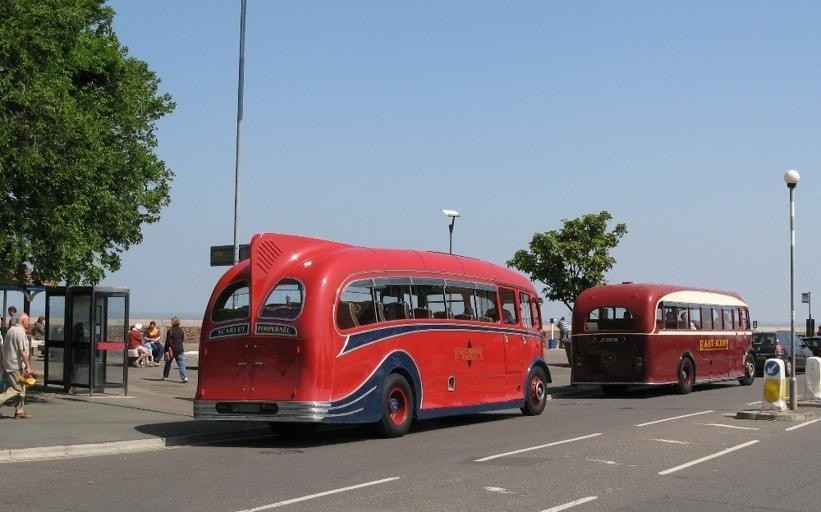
[339,300,495,330]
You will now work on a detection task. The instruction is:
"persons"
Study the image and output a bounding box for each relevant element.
[159,315,189,384]
[145,320,163,367]
[680,311,699,331]
[487,296,518,325]
[127,325,159,366]
[127,322,155,368]
[2,306,47,420]
[556,315,568,340]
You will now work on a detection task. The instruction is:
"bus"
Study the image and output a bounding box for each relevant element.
[191,231,554,442]
[566,280,758,395]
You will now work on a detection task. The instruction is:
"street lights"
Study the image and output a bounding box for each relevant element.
[781,167,803,412]
[438,206,462,256]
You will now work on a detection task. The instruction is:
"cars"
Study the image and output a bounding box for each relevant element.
[799,336,820,359]
[748,326,815,379]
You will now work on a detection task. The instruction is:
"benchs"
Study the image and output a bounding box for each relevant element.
[27,335,45,360]
[128,348,154,364]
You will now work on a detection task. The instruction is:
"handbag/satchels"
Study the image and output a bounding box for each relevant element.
[168,347,173,358]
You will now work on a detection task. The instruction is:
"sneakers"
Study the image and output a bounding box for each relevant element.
[162,378,168,382]
[183,377,188,382]
[15,413,31,418]
